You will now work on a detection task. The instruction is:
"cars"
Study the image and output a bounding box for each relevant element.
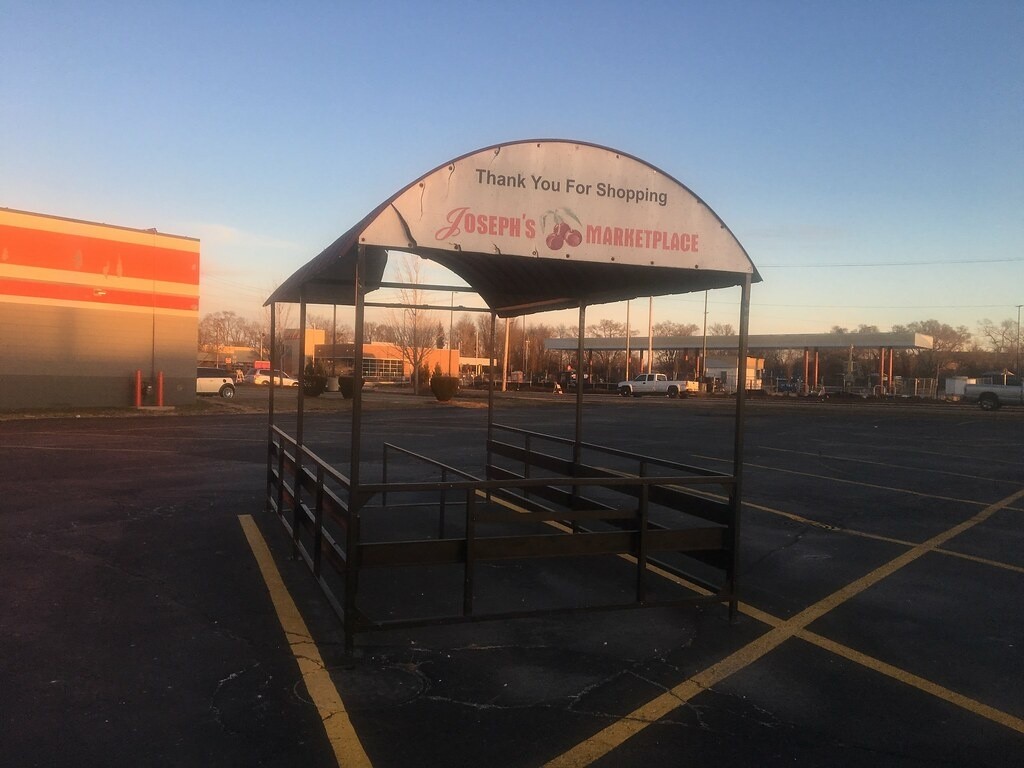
[243,368,298,388]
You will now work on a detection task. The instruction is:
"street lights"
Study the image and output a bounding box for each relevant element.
[1015,304,1023,386]
[448,291,460,377]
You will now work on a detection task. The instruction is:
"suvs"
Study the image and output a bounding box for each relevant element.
[196,366,235,400]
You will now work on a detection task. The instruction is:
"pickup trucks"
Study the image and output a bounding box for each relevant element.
[616,373,699,399]
[964,384,1024,411]
